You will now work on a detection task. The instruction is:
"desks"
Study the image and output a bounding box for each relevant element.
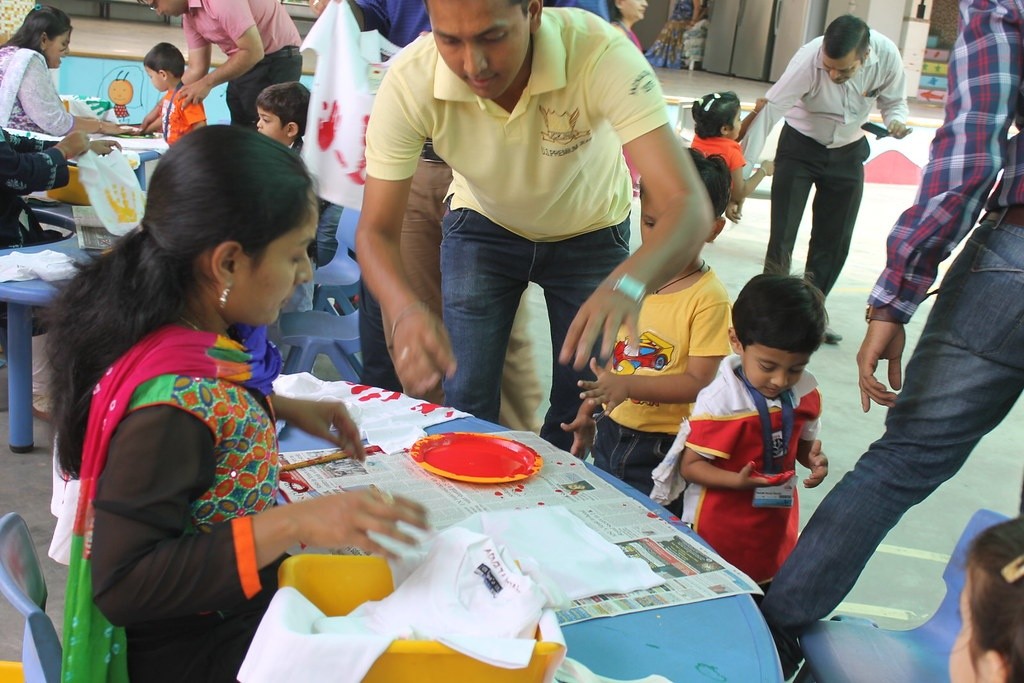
[0,189,158,455]
[3,123,169,245]
[266,376,783,683]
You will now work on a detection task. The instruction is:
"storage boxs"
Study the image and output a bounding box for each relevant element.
[277,550,567,683]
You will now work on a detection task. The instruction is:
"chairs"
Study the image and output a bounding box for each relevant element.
[0,512,69,683]
[793,508,1013,683]
[278,205,363,397]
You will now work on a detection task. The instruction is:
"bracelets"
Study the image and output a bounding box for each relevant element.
[760,167,767,176]
[389,300,426,349]
[93,118,103,133]
[866,305,903,323]
[752,110,758,114]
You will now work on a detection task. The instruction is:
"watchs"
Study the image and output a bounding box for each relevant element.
[600,273,646,308]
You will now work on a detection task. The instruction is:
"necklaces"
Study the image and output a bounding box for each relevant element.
[652,259,706,293]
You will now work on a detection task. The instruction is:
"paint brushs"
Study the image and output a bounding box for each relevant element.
[281,446,379,472]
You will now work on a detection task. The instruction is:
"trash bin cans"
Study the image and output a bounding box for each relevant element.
[279,553,559,683]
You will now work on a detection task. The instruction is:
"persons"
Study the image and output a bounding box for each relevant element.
[0,125,122,250]
[140,0,302,130]
[690,90,774,202]
[759,0,1024,683]
[561,148,733,514]
[0,5,139,137]
[30,123,431,683]
[546,0,648,196]
[949,514,1024,683]
[724,14,909,343]
[310,0,541,429]
[679,248,828,608]
[355,0,715,460]
[644,0,711,67]
[130,42,207,148]
[255,81,311,154]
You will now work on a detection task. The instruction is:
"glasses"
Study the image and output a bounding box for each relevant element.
[149,0,158,11]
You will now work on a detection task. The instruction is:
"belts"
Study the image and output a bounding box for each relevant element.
[264,48,299,58]
[420,140,444,162]
[979,205,1024,229]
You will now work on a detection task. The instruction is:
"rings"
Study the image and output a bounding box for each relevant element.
[381,490,393,503]
